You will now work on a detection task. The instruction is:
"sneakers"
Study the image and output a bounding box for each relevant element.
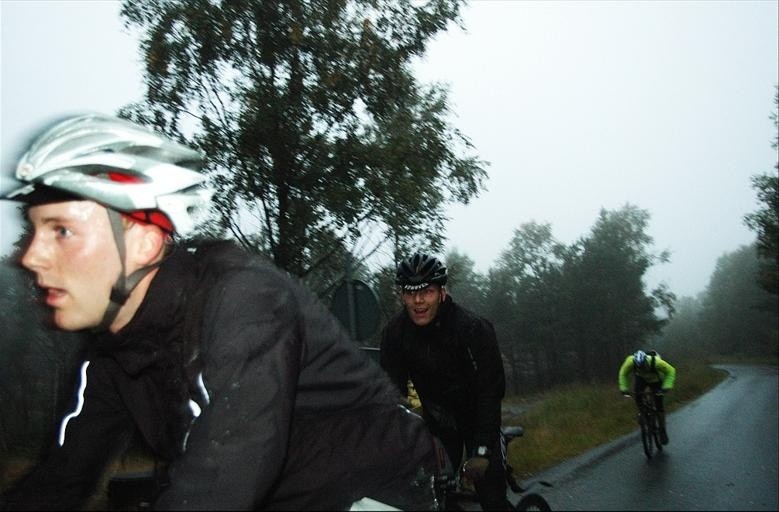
[658,426,668,445]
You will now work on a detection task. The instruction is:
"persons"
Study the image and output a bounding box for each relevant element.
[618,348,676,444]
[376,253,510,510]
[1,116,451,511]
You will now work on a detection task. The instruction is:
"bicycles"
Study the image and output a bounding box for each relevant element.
[630,392,663,461]
[396,404,553,512]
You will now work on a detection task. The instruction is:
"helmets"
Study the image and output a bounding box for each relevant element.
[634,350,647,368]
[394,252,448,289]
[1,116,216,241]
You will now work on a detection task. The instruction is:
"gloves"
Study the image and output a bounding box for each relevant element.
[656,389,665,398]
[460,457,489,486]
